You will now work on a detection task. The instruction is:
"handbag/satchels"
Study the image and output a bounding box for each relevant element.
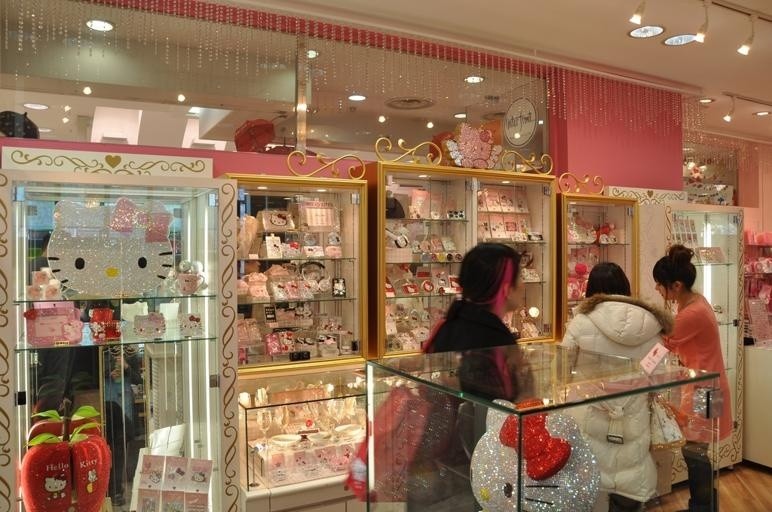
[344,376,464,506]
[648,389,689,452]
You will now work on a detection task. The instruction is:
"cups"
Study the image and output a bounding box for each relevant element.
[179,274,204,296]
[25,271,61,300]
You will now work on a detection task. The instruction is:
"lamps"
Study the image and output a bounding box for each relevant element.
[627,0,759,55]
[722,96,737,122]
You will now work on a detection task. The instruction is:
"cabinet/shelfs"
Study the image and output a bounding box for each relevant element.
[639,203,744,485]
[0,169,238,512]
[225,174,368,374]
[744,243,772,339]
[352,157,556,359]
[557,192,639,341]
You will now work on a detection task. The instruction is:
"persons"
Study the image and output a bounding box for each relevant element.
[28,234,141,505]
[654,244,734,512]
[553,262,673,512]
[407,240,537,511]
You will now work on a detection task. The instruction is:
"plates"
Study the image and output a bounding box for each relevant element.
[271,424,362,447]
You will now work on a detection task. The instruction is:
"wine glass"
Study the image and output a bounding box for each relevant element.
[257,410,271,450]
[274,406,288,434]
[330,399,346,424]
